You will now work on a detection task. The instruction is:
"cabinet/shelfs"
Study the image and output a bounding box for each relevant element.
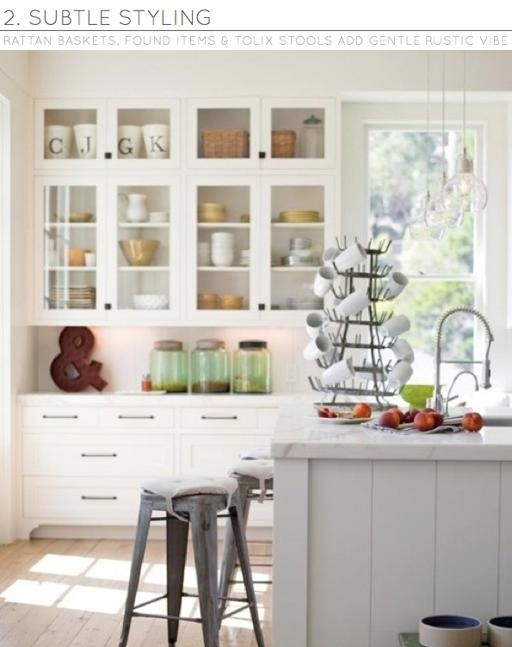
[29,98,181,172]
[27,173,182,328]
[182,173,340,328]
[17,392,176,545]
[181,99,337,173]
[176,391,316,530]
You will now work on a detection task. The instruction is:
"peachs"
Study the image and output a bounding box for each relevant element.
[460,413,482,431]
[351,402,371,418]
[378,405,444,431]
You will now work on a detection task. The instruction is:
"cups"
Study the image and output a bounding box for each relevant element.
[45,124,73,158]
[302,241,415,390]
[118,125,142,157]
[142,123,170,158]
[73,123,97,159]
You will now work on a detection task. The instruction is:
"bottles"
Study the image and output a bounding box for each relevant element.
[140,374,152,392]
[232,340,271,394]
[298,115,324,158]
[190,338,231,394]
[149,340,189,393]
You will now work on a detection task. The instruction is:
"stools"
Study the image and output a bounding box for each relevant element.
[118,476,265,647]
[218,460,273,628]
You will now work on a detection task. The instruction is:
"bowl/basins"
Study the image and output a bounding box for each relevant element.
[399,384,435,407]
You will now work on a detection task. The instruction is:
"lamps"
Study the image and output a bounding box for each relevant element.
[440,50,489,213]
[408,50,446,242]
[424,50,465,229]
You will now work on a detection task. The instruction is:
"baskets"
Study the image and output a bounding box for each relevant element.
[203,130,248,158]
[272,130,296,158]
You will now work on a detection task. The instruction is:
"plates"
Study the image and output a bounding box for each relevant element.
[314,413,374,424]
[46,187,325,311]
